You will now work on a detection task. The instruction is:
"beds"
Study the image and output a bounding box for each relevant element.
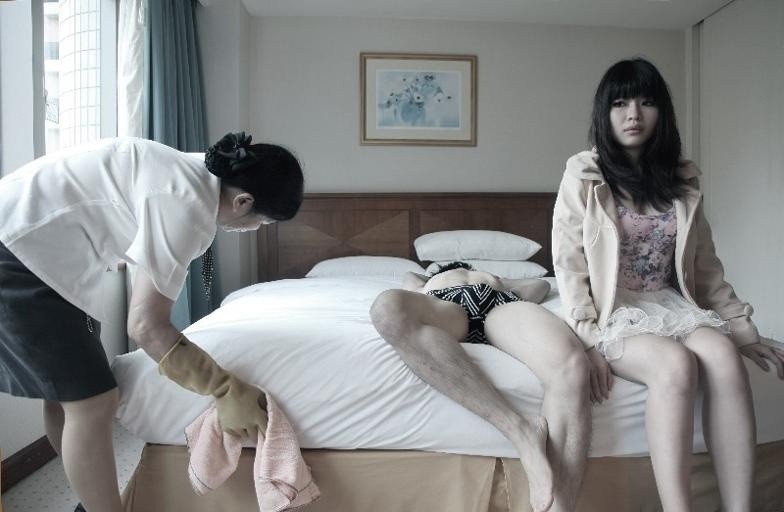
[116,279,784,512]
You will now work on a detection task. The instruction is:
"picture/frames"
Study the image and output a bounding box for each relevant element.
[359,50,478,146]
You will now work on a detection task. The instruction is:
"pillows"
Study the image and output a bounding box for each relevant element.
[303,229,551,282]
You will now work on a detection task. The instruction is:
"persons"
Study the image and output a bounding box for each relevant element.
[0,132,305,510]
[370,258,596,511]
[549,56,783,512]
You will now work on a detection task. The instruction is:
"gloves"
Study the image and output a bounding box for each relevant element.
[158,332,268,443]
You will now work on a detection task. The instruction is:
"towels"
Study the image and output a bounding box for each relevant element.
[174,391,322,511]
